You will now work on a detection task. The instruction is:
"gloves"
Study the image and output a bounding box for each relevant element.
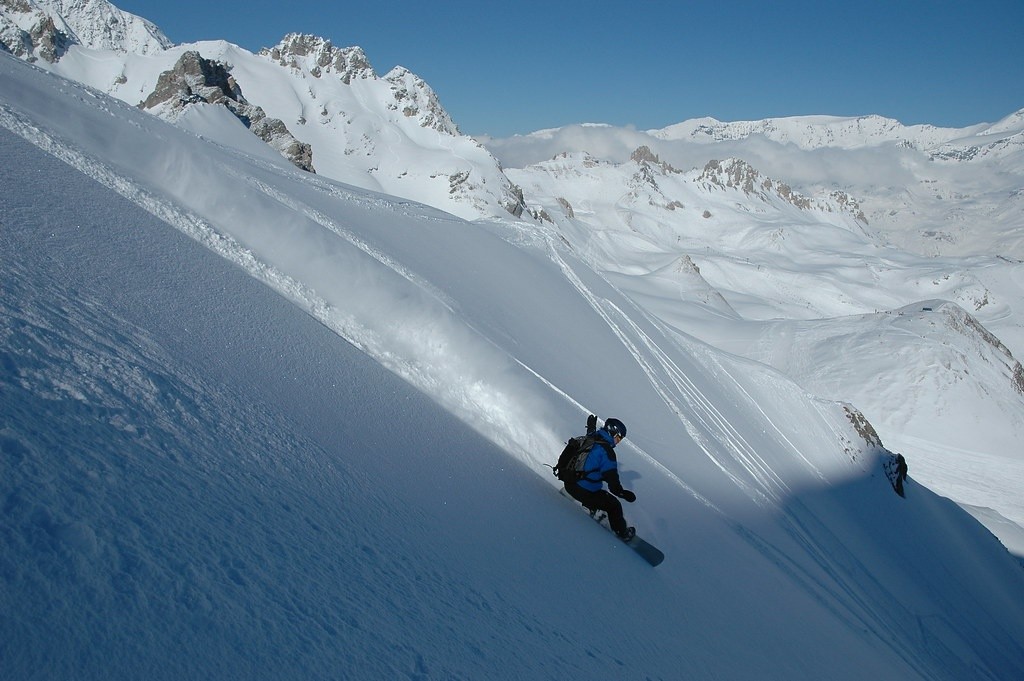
[616,490,636,502]
[585,415,597,429]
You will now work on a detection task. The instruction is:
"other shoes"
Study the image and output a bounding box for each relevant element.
[627,527,636,538]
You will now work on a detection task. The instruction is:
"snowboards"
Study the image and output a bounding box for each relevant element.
[560,485,665,567]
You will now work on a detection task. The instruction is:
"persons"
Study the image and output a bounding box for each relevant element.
[564,418,636,541]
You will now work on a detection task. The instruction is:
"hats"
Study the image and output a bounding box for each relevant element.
[605,418,626,437]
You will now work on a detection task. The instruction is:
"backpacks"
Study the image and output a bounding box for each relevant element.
[552,431,608,482]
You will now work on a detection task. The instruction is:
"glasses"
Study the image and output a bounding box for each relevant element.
[613,426,624,440]
